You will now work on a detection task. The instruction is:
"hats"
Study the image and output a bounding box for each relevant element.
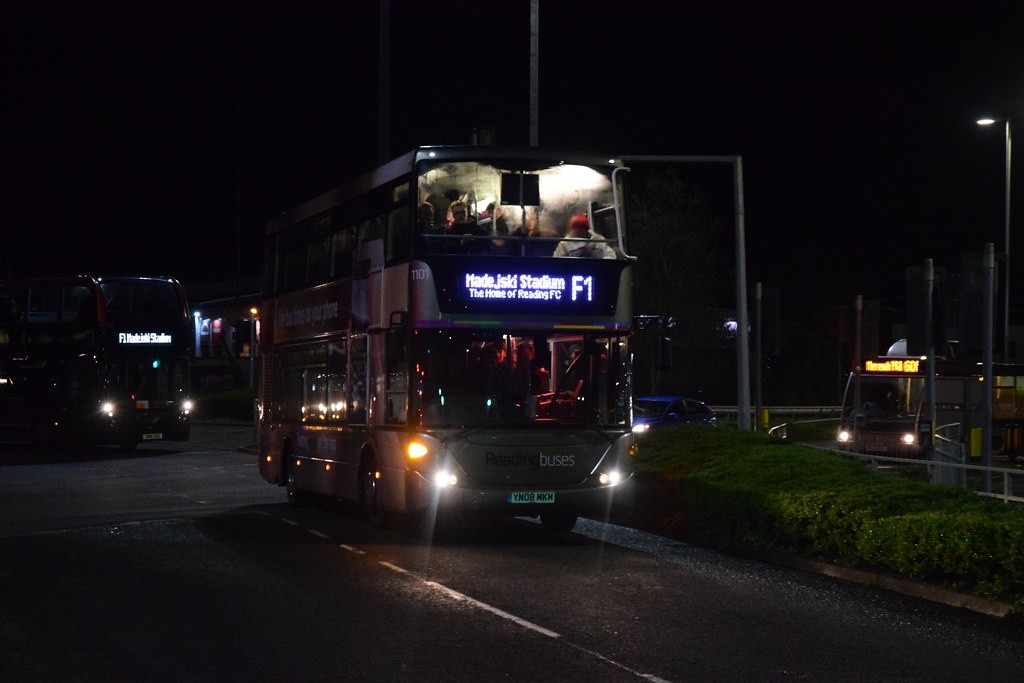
[570,215,588,230]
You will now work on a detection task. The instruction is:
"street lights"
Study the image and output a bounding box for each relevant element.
[975,117,1014,364]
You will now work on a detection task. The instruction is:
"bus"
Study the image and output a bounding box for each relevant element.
[0,273,194,459]
[835,356,981,460]
[256,144,644,536]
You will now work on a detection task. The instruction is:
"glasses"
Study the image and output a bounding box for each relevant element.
[453,210,467,215]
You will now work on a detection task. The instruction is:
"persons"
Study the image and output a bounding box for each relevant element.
[477,340,589,421]
[394,186,617,260]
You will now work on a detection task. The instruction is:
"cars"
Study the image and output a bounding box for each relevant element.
[633,395,718,435]
